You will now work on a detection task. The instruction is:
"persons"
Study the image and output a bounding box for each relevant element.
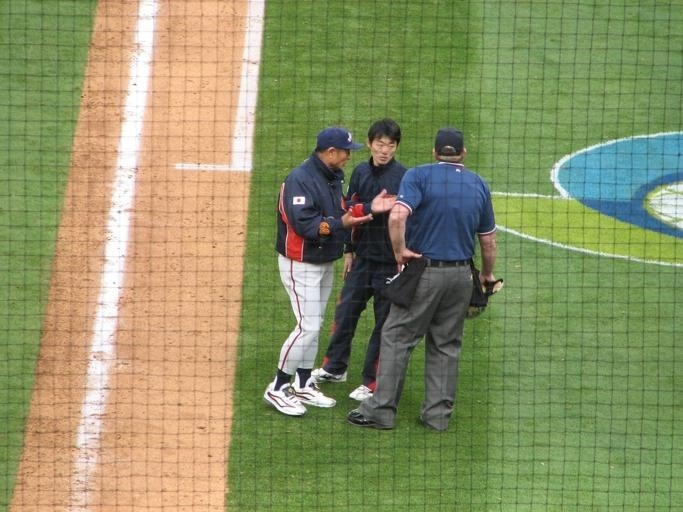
[348,128,496,429]
[264,129,397,415]
[311,119,408,402]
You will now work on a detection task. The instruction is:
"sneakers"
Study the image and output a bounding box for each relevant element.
[349,384,374,401]
[310,367,347,382]
[264,383,307,416]
[346,408,394,429]
[291,376,337,408]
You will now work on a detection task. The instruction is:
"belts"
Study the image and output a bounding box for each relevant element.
[424,258,471,267]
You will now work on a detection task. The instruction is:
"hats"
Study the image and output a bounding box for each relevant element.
[435,128,463,155]
[317,128,364,149]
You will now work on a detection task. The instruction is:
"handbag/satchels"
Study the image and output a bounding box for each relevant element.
[470,257,488,305]
[381,258,427,309]
